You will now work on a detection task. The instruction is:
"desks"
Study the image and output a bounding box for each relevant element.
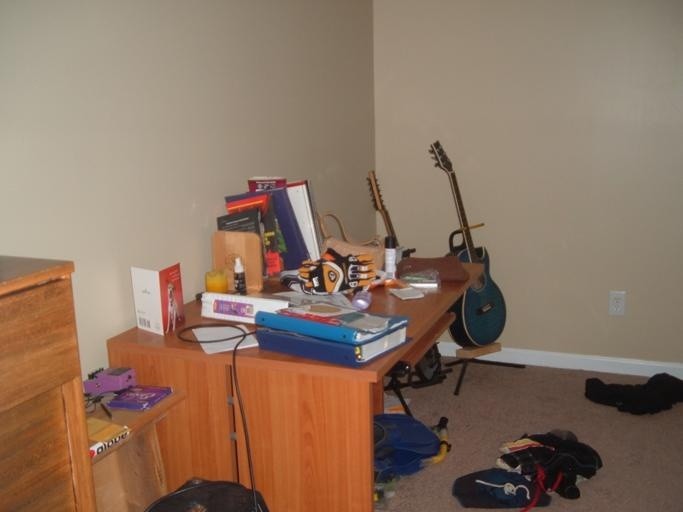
[80,255,486,512]
[82,385,186,511]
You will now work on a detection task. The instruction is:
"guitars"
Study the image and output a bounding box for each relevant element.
[368,170,442,382]
[428,141,507,348]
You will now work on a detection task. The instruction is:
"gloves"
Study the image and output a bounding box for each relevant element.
[298,248,377,293]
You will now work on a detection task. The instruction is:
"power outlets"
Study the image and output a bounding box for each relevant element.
[608,290,626,317]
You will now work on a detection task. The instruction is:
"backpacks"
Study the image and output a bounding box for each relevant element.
[372,412,439,481]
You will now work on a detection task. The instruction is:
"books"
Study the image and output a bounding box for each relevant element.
[217,176,320,279]
[86,415,131,458]
[131,262,185,334]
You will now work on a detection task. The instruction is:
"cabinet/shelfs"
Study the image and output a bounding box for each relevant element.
[0,253,94,512]
